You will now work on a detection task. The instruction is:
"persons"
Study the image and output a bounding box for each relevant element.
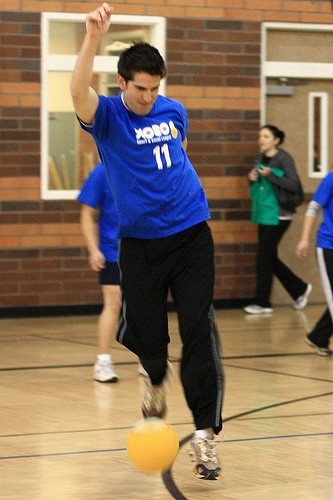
[76,160,175,384]
[294,171,333,356]
[70,2,226,482]
[243,125,313,314]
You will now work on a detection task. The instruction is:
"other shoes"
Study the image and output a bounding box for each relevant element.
[293,282,312,310]
[244,305,273,314]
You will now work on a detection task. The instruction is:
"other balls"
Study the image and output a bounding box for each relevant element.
[125,418,179,472]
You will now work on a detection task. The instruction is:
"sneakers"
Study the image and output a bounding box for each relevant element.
[141,380,168,420]
[93,360,119,383]
[137,360,174,379]
[189,430,221,480]
[304,335,333,356]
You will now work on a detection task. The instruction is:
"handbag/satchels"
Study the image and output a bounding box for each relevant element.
[274,151,306,208]
